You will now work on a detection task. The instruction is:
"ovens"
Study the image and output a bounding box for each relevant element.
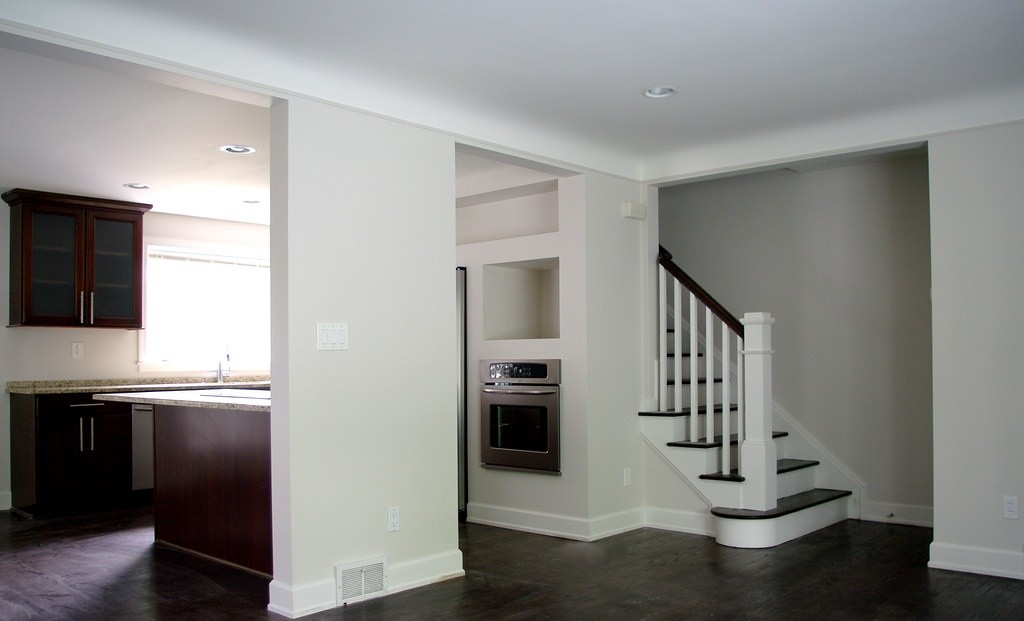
[478,359,562,476]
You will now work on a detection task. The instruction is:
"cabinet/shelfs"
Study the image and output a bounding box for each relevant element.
[1,187,153,333]
[10,392,134,524]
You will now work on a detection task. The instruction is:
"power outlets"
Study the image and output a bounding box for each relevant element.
[1003,495,1018,520]
[72,343,83,360]
